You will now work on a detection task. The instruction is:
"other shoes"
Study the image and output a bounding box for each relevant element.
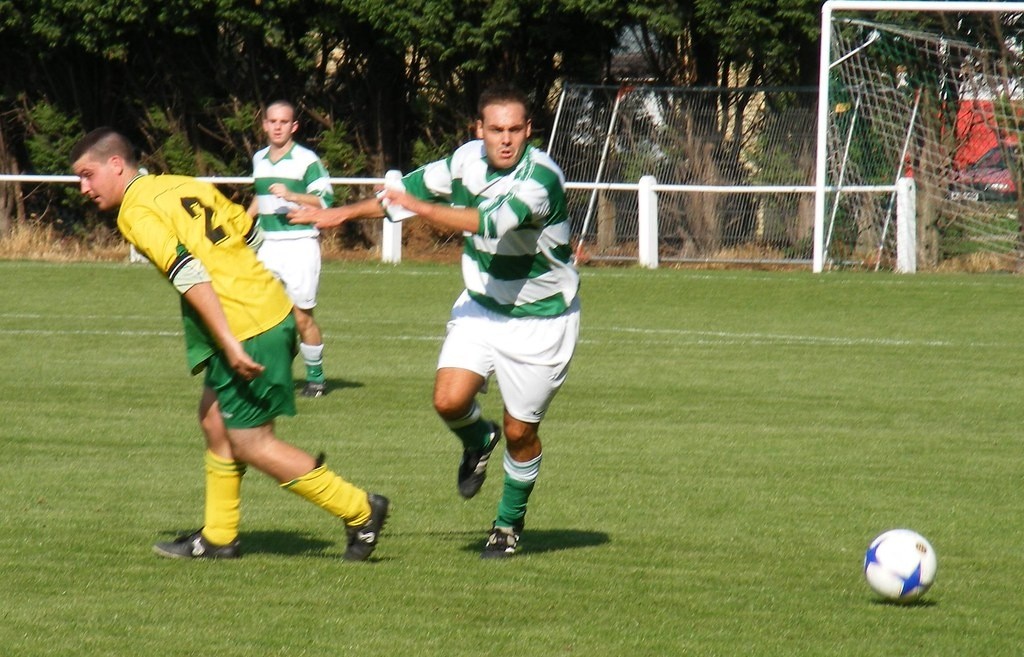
[299,379,328,398]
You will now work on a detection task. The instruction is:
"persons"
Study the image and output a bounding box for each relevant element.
[287,89,582,561]
[245,100,335,398]
[205,161,239,202]
[69,126,392,561]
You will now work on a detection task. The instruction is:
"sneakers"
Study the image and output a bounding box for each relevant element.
[458,420,502,499]
[345,492,389,561]
[152,526,241,559]
[480,520,525,559]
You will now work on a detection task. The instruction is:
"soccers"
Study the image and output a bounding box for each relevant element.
[860,526,940,604]
